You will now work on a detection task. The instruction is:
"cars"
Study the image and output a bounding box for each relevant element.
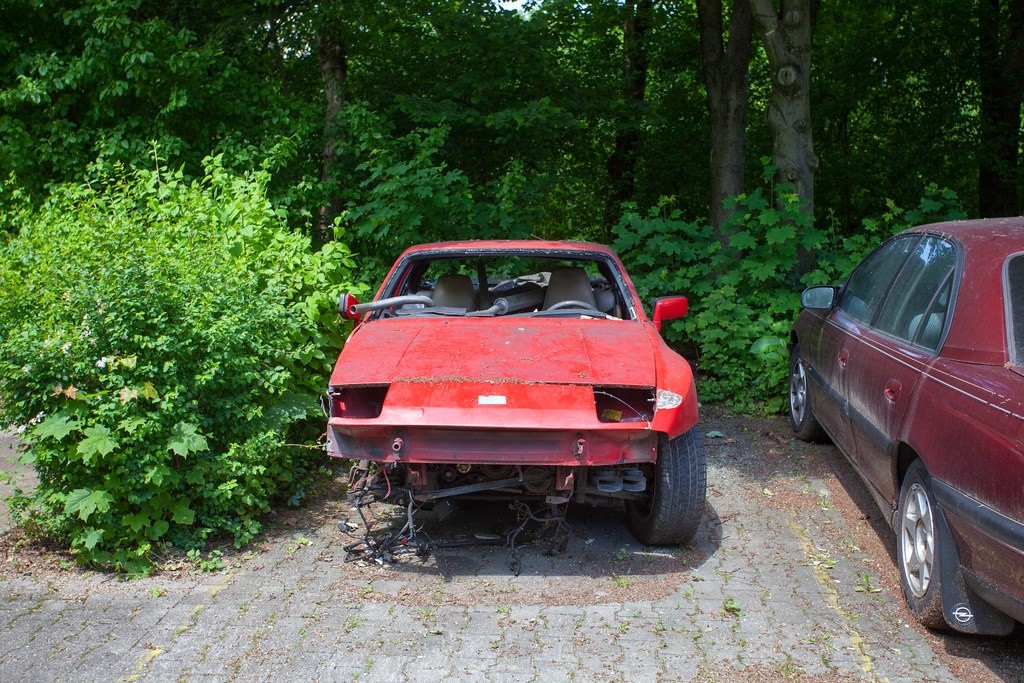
[782,212,1023,629]
[319,239,713,550]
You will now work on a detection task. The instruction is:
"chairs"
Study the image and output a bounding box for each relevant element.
[542,267,599,312]
[431,274,478,311]
[908,313,946,344]
